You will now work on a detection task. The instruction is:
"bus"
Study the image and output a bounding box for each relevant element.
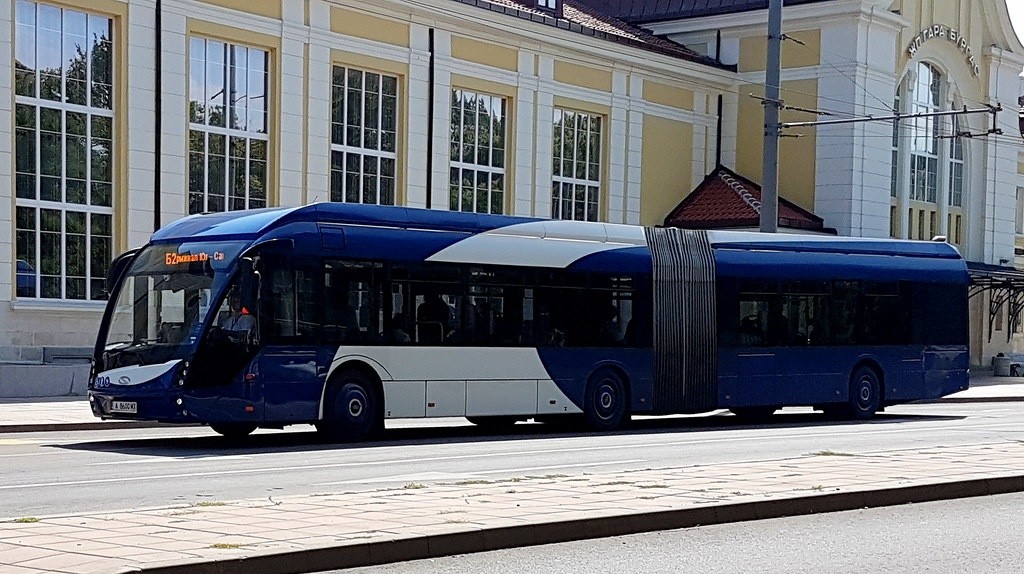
[17,261,50,296]
[88,197,974,441]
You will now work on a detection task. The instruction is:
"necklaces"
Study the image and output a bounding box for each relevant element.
[231,314,242,330]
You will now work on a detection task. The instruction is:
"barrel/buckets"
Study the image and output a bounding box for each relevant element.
[994,357,1010,376]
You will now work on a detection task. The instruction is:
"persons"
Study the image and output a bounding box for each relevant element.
[219,292,257,341]
[740,302,836,347]
[385,291,624,347]
